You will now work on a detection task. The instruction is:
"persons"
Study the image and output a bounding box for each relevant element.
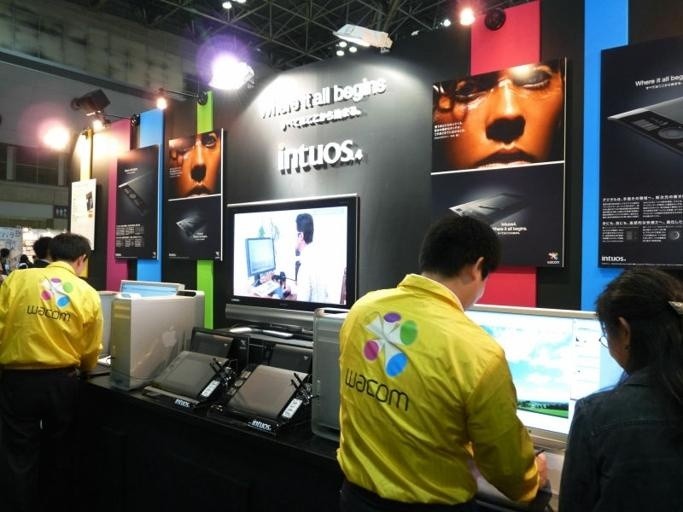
[336,216,548,512]
[294,212,326,302]
[167,131,221,197]
[0,233,103,512]
[558,266,683,512]
[432,63,565,170]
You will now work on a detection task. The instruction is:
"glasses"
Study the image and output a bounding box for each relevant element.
[599,335,609,348]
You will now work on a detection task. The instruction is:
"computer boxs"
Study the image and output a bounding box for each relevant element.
[312,307,352,441]
[109,290,206,391]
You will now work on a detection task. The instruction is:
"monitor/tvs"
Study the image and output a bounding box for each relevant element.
[463,304,629,452]
[224,192,362,313]
[245,237,275,287]
[119,279,185,295]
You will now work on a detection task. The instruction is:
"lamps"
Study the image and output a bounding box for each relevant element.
[155,87,208,111]
[334,23,393,49]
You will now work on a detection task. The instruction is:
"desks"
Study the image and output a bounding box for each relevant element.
[83,351,566,512]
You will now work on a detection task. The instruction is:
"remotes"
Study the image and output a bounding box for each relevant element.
[122,185,146,216]
